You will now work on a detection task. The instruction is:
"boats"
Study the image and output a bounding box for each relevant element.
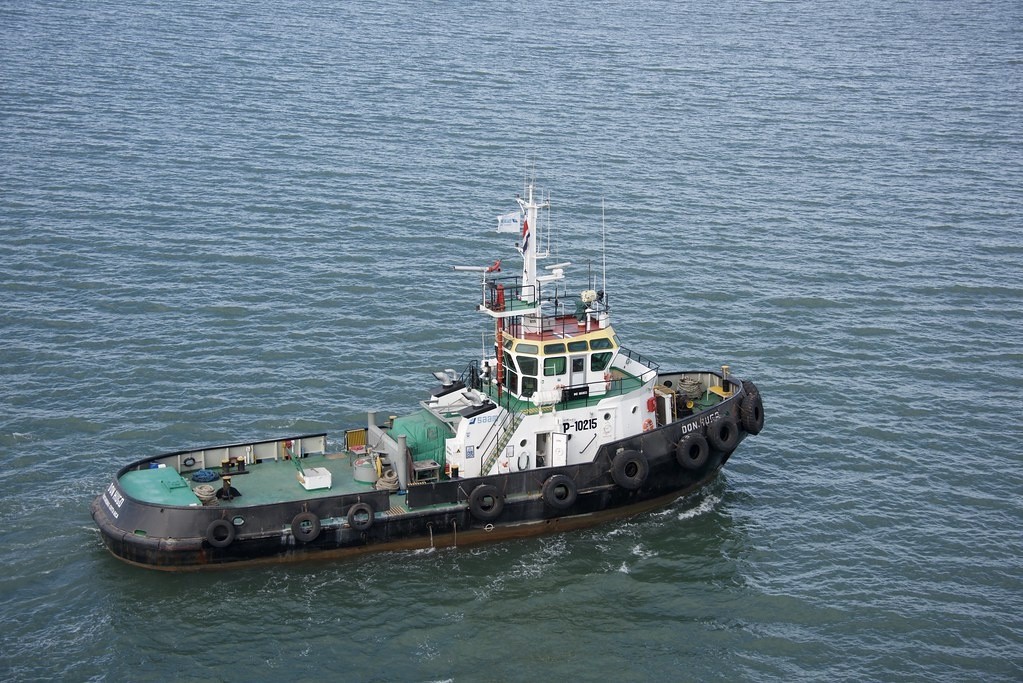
[88,175,764,575]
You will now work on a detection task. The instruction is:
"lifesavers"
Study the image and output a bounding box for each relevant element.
[605,373,611,390]
[675,432,710,471]
[468,484,505,522]
[541,473,577,510]
[643,418,654,432]
[346,502,376,532]
[206,519,236,548]
[610,449,650,490]
[741,380,759,396]
[707,415,739,452]
[290,511,322,542]
[740,395,764,436]
